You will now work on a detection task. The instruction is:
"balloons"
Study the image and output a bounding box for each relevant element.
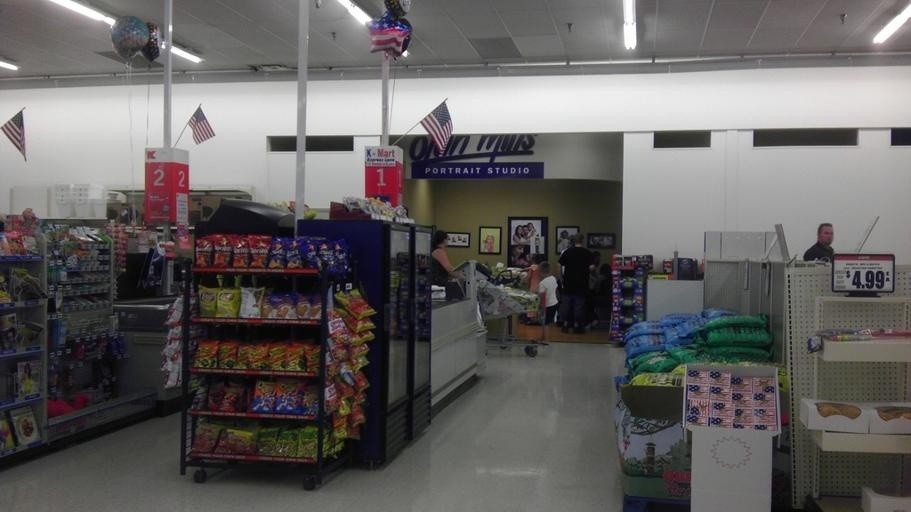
[367,12,411,56]
[398,17,412,56]
[147,22,163,62]
[110,16,151,57]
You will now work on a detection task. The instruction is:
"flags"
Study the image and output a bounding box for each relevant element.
[189,106,215,145]
[420,102,457,159]
[1,111,30,162]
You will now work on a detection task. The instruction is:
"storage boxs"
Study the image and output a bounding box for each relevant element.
[53,184,128,218]
[862,485,911,512]
[798,398,911,436]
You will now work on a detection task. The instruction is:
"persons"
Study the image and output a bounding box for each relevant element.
[803,223,834,261]
[559,231,570,252]
[483,236,496,252]
[558,233,596,334]
[538,262,558,338]
[529,255,546,297]
[560,235,573,287]
[586,264,613,329]
[590,251,602,288]
[433,231,465,280]
[513,223,539,244]
[591,234,615,247]
[511,246,528,266]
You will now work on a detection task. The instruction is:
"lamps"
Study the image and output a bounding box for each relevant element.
[623,0,636,51]
[0,58,21,73]
[48,0,204,63]
[332,0,410,59]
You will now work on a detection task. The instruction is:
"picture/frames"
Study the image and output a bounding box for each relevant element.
[555,224,580,255]
[586,232,615,248]
[479,226,502,255]
[445,232,471,248]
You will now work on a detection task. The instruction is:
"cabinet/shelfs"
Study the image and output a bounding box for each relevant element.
[180,256,358,489]
[646,279,704,321]
[811,296,911,512]
[1,233,47,458]
[48,218,115,428]
